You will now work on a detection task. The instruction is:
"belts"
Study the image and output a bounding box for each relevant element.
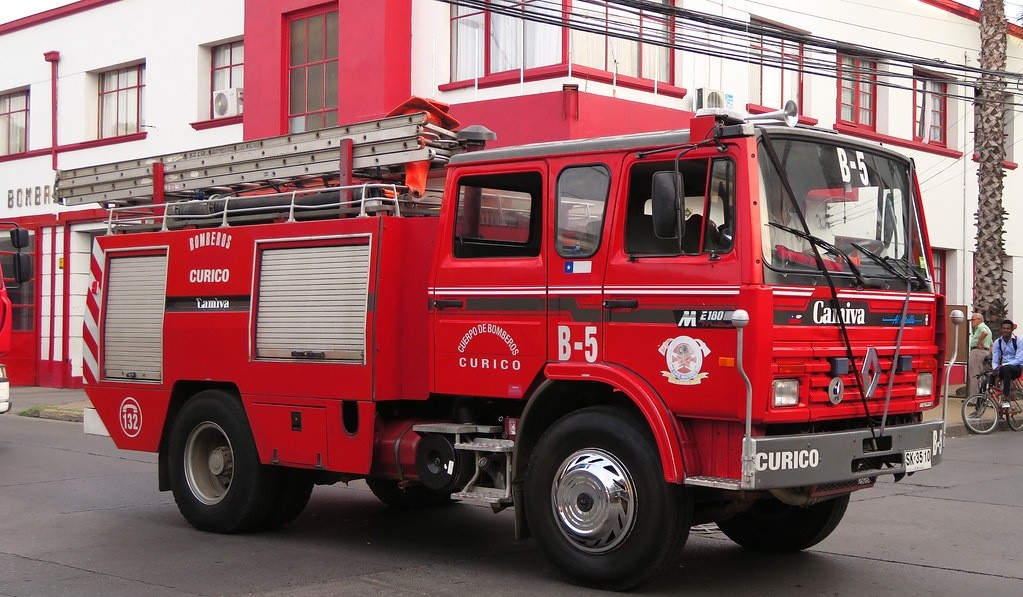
[970,346,987,350]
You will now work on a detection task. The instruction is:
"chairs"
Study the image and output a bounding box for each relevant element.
[683,214,718,252]
[587,214,651,254]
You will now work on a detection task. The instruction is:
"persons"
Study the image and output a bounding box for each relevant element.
[962,313,993,406]
[768,181,835,257]
[557,202,593,253]
[970,319,1023,416]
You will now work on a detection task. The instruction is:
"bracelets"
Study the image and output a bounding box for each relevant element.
[575,241,581,251]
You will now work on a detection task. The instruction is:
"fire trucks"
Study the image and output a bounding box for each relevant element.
[0,221,34,415]
[53,95,963,591]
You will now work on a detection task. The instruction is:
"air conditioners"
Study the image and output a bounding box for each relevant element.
[693,86,733,116]
[212,87,243,119]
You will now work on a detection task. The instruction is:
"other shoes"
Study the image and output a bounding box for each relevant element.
[966,412,982,420]
[1002,402,1011,409]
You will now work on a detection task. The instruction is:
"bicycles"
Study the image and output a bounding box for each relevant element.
[972,356,1023,407]
[962,367,1023,435]
